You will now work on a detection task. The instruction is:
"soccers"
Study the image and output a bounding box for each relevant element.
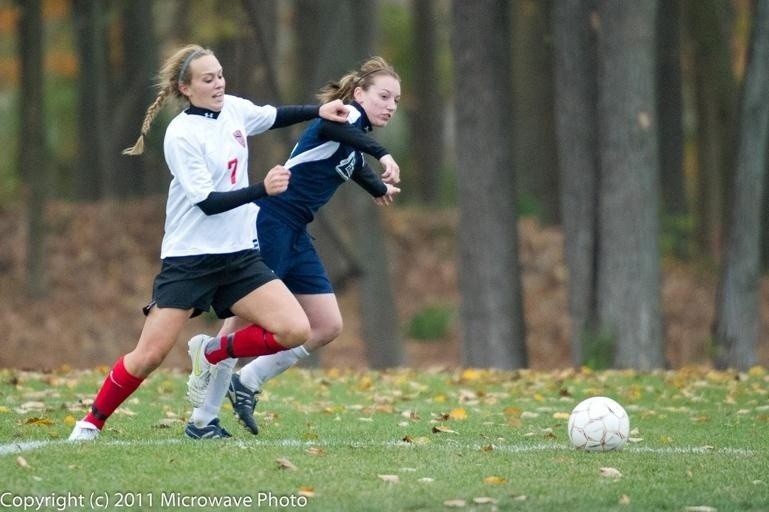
[568,397,629,452]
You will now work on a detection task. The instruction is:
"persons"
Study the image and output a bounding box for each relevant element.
[68,43,350,441]
[184,55,408,440]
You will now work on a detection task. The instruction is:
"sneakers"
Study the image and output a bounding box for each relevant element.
[185,418,232,439]
[67,414,100,441]
[225,372,258,434]
[186,334,218,408]
